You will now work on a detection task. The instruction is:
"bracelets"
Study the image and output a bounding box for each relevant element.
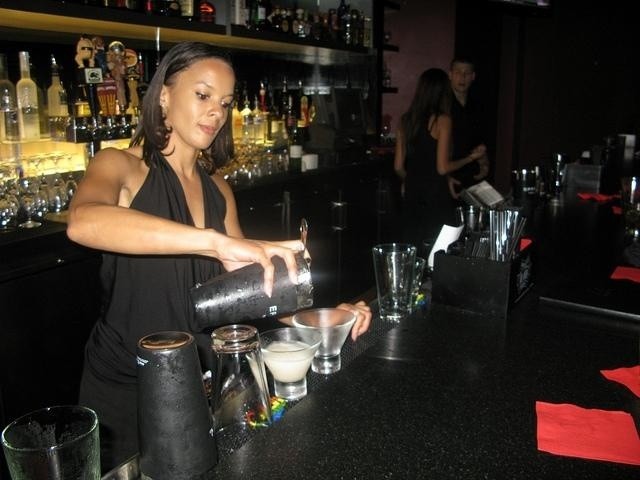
[469,154,476,164]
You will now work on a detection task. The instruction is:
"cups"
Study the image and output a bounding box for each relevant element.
[187,250,315,329]
[371,242,416,324]
[135,332,218,479]
[291,306,355,375]
[1,405,101,479]
[622,177,640,268]
[413,255,425,296]
[258,327,323,405]
[458,206,484,235]
[210,325,274,455]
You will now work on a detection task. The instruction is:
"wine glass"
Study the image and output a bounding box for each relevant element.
[0,150,78,233]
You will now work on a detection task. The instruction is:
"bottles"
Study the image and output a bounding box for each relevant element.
[0,0,215,144]
[229,0,392,184]
[551,154,562,195]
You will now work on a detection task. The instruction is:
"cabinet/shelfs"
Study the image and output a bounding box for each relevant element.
[379,1,400,156]
[0,7,380,423]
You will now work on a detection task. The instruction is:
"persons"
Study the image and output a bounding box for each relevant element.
[393,66,486,252]
[445,56,495,201]
[66,40,374,476]
